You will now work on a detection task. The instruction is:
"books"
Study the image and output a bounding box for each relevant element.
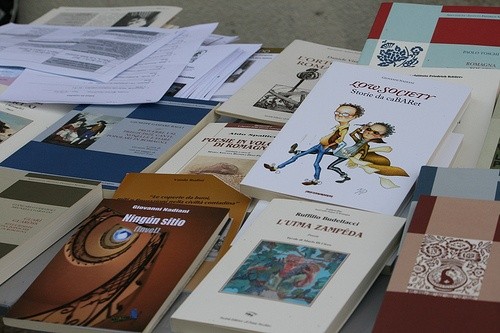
[155,122,284,219]
[215,40,362,125]
[169,198,405,333]
[396,165,500,257]
[112,173,253,317]
[238,61,471,218]
[0,96,221,198]
[356,67,499,168]
[372,194,500,333]
[0,167,104,287]
[2,198,230,333]
[355,2,499,69]
